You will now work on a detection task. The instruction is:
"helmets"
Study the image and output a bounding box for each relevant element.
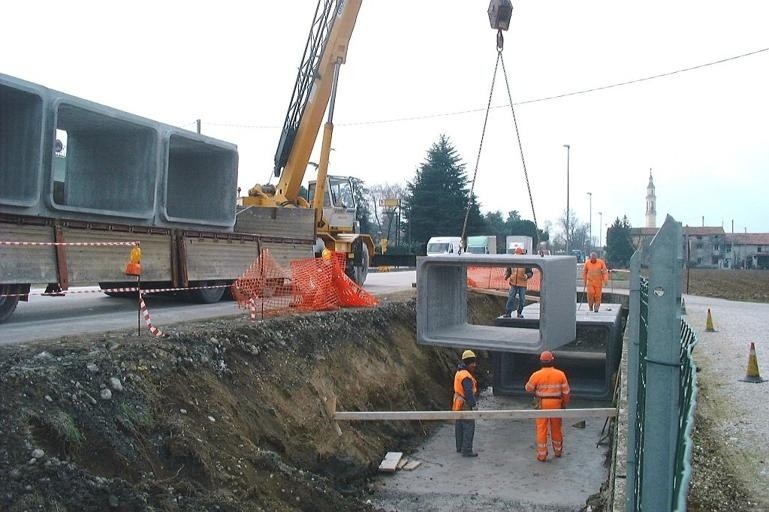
[540,351,554,360]
[462,350,476,360]
[591,253,596,259]
[515,248,523,253]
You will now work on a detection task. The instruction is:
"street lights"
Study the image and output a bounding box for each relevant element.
[562,144,570,257]
[586,191,592,257]
[598,211,602,259]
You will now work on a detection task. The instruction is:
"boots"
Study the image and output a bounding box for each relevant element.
[503,310,523,318]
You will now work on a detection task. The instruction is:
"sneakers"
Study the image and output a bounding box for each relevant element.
[457,448,478,456]
[589,304,598,312]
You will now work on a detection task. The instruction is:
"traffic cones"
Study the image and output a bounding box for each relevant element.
[737,342,769,383]
[702,308,720,332]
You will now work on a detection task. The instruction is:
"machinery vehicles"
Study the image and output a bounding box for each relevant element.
[241,0,515,290]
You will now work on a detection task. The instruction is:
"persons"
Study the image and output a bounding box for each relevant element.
[502,246,533,317]
[582,251,609,312]
[449,350,481,457]
[525,350,572,462]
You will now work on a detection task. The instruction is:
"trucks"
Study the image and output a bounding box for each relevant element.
[505,235,533,256]
[466,234,497,254]
[426,235,464,257]
